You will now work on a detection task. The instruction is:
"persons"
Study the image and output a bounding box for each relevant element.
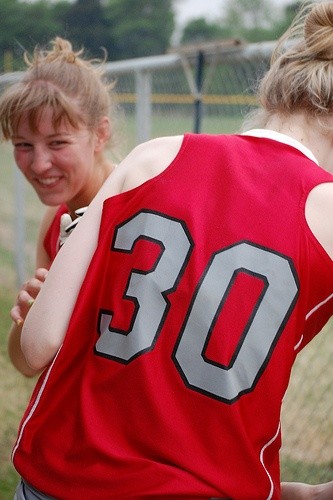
[1,37,121,380]
[7,1,333,500]
